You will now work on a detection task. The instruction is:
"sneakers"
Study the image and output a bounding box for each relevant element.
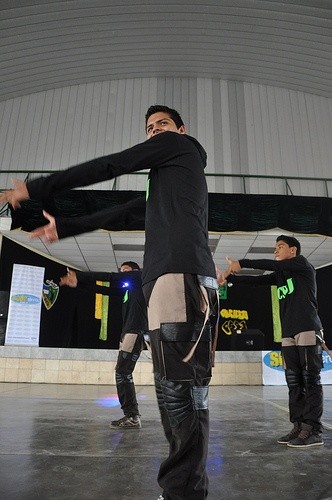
[276,422,303,444]
[287,423,324,447]
[109,415,141,429]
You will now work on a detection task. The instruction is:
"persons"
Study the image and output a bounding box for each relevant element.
[61,261,148,429]
[216,235,324,448]
[0,105,219,500]
